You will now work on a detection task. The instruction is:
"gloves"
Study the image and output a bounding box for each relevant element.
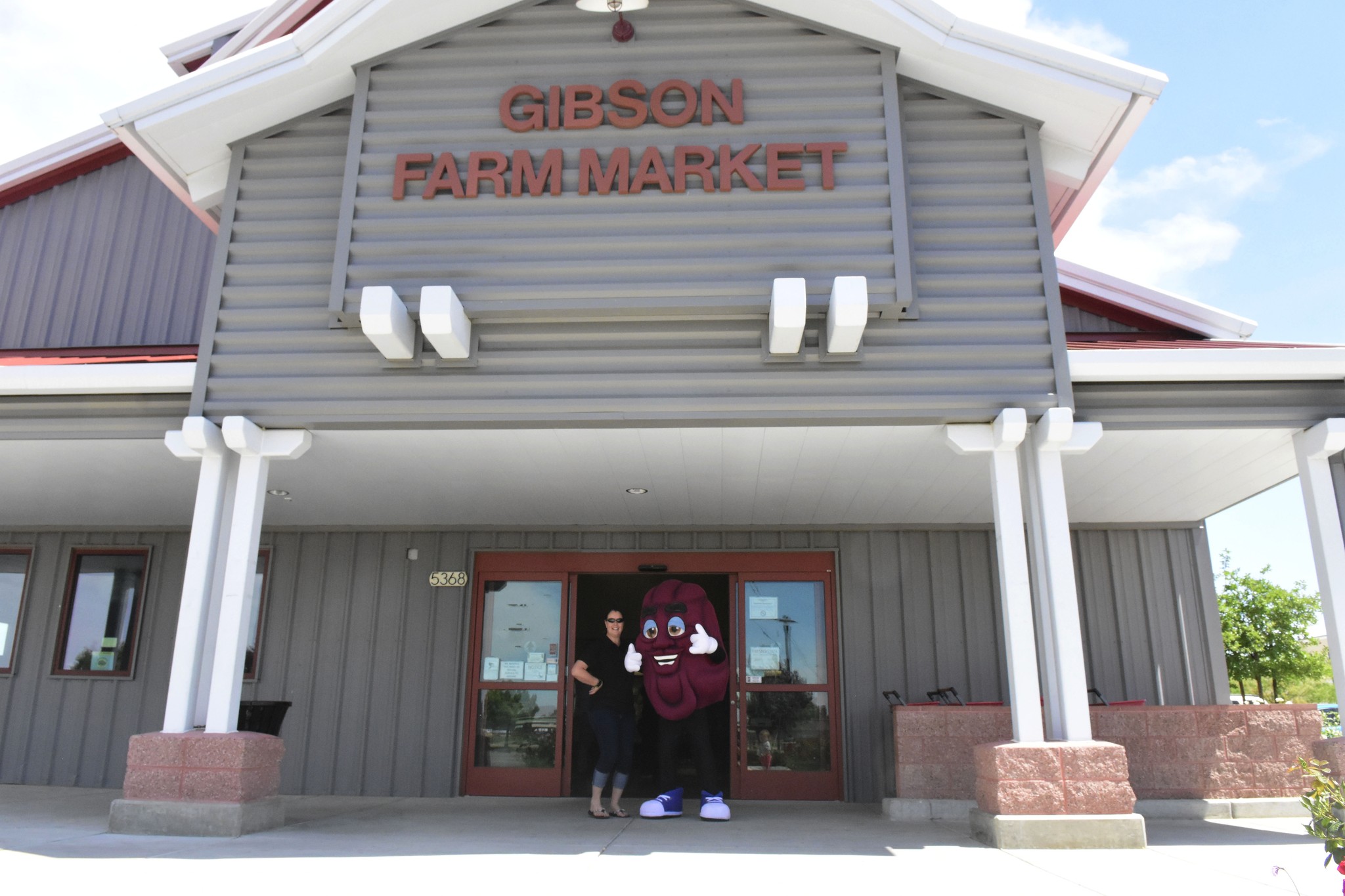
[624,642,642,672]
[689,624,718,655]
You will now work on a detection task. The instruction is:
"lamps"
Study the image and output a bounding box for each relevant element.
[576,0,649,43]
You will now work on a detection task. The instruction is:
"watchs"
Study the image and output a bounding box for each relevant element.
[592,678,602,688]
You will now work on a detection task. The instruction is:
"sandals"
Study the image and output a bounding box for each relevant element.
[588,808,609,819]
[609,808,630,818]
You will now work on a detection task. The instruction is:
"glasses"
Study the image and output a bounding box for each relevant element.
[642,603,687,616]
[607,617,623,623]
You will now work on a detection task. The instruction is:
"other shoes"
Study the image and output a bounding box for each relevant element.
[640,788,684,818]
[699,790,731,821]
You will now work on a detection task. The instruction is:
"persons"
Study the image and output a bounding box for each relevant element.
[571,606,634,818]
[756,730,772,770]
[624,578,730,822]
